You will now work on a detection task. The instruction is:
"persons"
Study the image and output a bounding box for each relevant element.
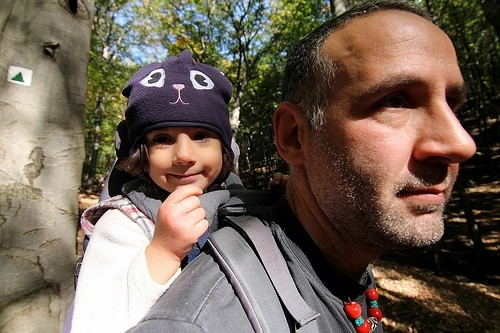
[124,0,477,333]
[61,47,291,332]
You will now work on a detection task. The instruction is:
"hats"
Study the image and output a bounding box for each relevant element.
[122,50,234,149]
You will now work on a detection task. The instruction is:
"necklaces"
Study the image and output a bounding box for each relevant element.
[336,267,384,333]
